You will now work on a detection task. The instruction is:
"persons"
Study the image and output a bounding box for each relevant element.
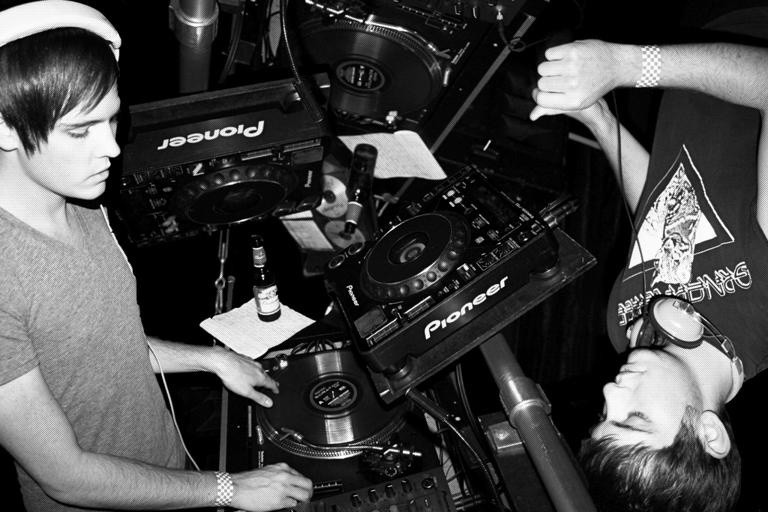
[528,0,768,511]
[0,1,315,512]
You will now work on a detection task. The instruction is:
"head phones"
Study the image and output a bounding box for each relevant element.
[0,0,123,65]
[625,296,745,405]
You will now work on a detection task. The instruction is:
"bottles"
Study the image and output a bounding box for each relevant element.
[249,235,281,322]
[343,145,377,234]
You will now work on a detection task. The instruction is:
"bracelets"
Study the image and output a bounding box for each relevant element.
[634,43,662,89]
[211,470,233,507]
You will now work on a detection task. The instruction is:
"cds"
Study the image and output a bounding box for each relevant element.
[326,219,365,256]
[316,176,349,221]
